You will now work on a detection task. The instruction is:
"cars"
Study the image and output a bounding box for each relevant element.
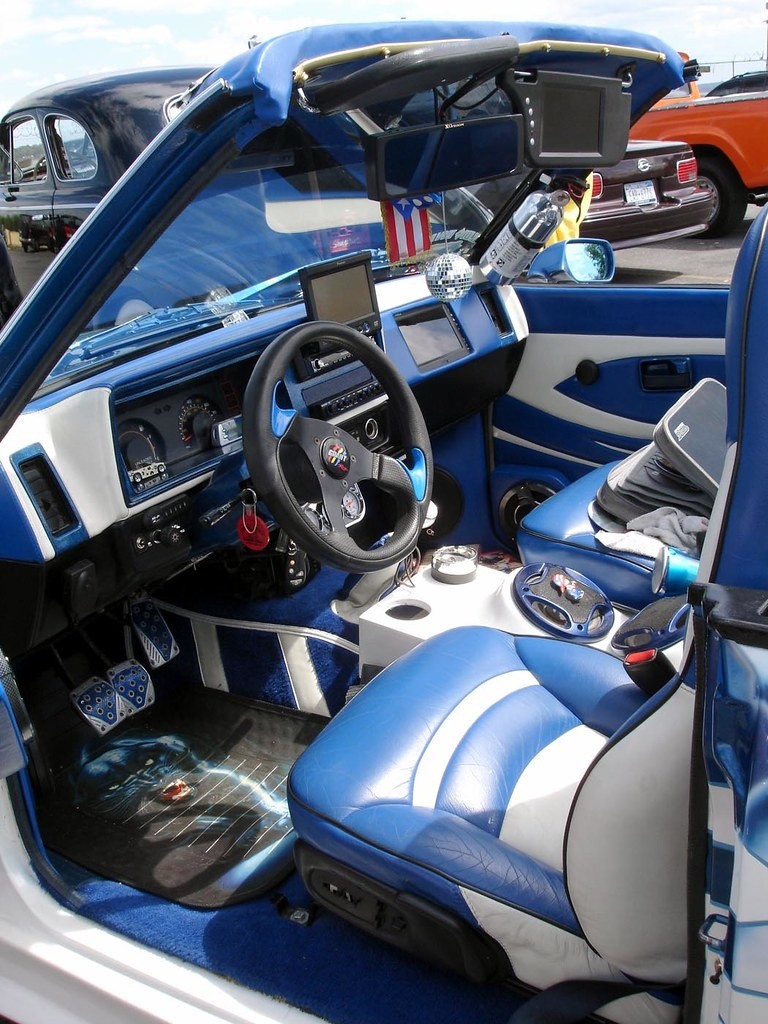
[0,16,768,1024]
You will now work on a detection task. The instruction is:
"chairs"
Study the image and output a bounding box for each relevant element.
[285,193,768,1024]
